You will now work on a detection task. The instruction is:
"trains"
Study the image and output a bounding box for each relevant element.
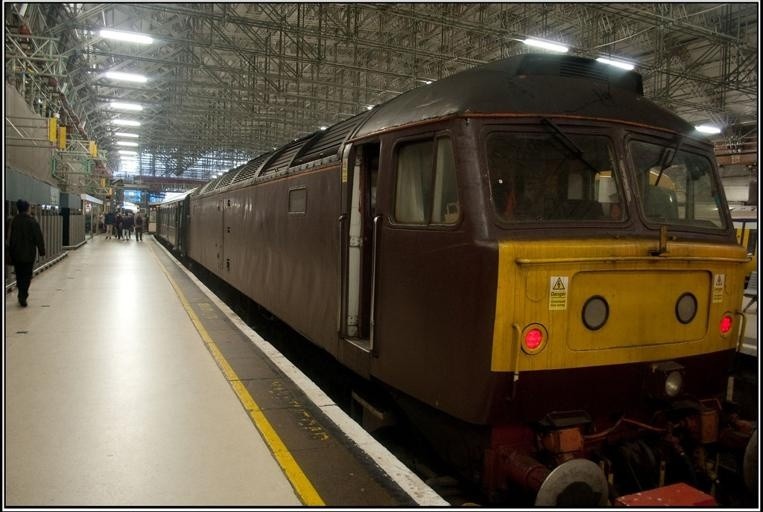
[590,161,681,222]
[696,200,757,291]
[144,51,752,510]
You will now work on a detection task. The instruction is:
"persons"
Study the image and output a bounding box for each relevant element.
[4,199,46,308]
[99,209,149,241]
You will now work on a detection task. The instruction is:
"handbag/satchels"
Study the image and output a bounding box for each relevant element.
[5,220,14,266]
[129,225,134,232]
[137,215,143,225]
